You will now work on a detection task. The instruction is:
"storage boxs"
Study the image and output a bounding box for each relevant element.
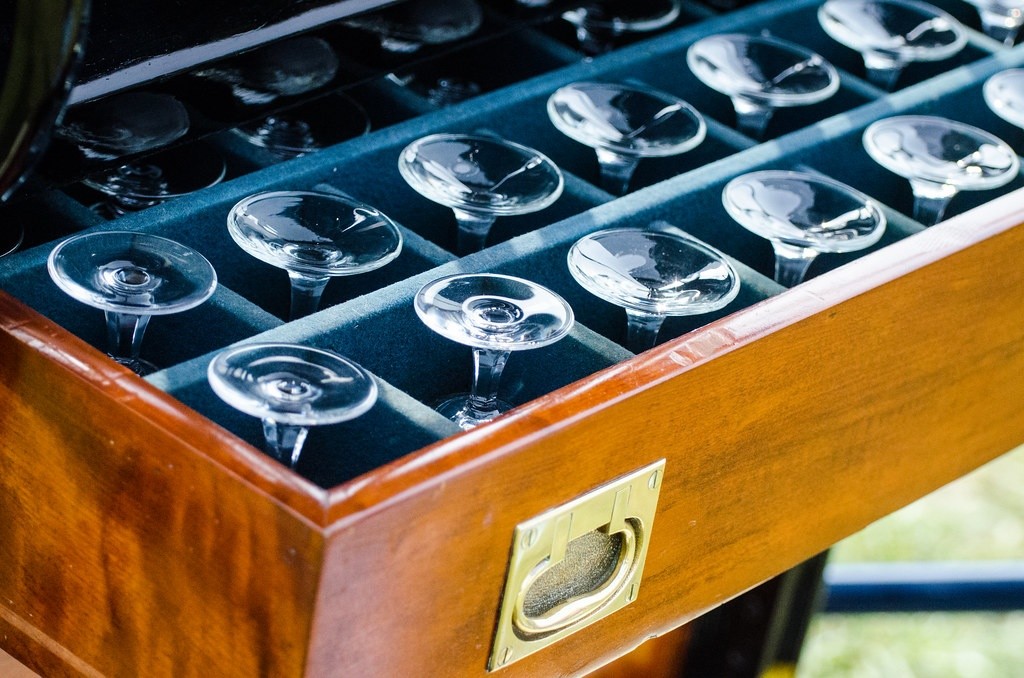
[0,0,1024,678]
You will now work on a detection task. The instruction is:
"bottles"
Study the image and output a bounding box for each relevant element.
[225,188,403,326]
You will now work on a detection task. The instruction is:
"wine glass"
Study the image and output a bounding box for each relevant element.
[411,270,575,438]
[861,114,1021,232]
[566,228,740,359]
[545,81,707,199]
[397,132,564,258]
[684,33,841,142]
[43,1,700,210]
[207,339,379,484]
[817,0,969,95]
[46,230,219,387]
[720,169,886,295]
